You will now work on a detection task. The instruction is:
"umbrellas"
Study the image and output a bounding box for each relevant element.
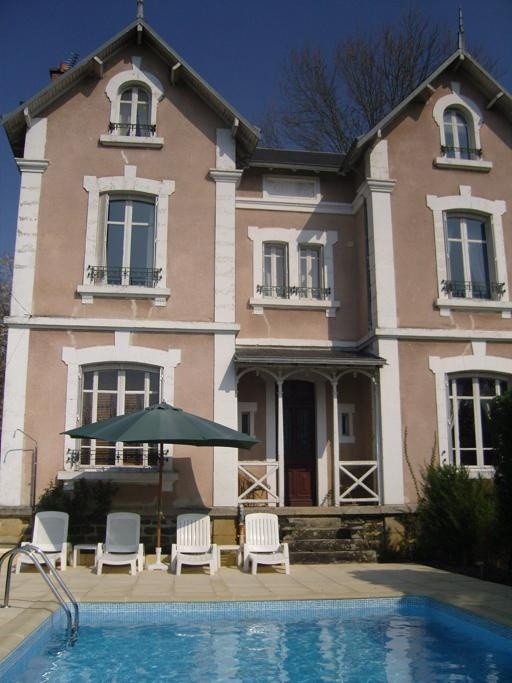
[56,400,262,561]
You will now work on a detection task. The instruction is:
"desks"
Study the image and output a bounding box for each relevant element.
[72,543,98,568]
[218,544,241,568]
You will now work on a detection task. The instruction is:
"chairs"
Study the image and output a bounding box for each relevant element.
[171,512,218,576]
[15,511,72,574]
[97,511,147,575]
[244,513,290,575]
